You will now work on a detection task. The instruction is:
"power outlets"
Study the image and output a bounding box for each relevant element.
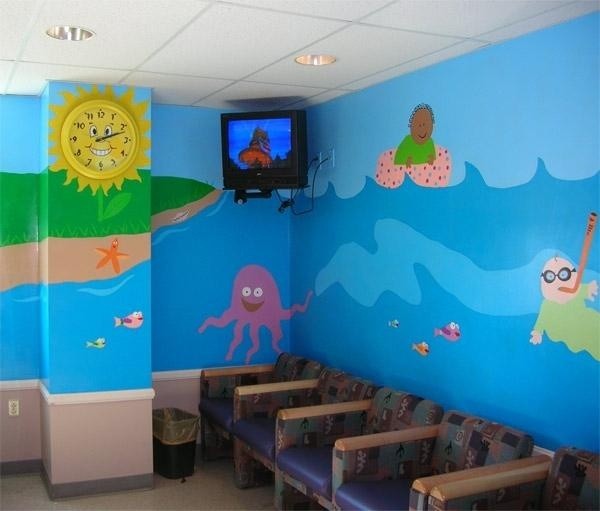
[8,399,19,416]
[310,152,322,171]
[324,149,334,168]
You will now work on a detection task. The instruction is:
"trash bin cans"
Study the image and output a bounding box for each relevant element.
[152,407,200,479]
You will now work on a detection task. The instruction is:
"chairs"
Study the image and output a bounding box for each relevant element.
[236,366,379,490]
[426,447,599,510]
[335,409,550,510]
[199,352,326,490]
[276,387,446,511]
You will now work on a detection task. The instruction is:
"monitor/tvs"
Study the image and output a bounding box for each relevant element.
[221,109,309,190]
